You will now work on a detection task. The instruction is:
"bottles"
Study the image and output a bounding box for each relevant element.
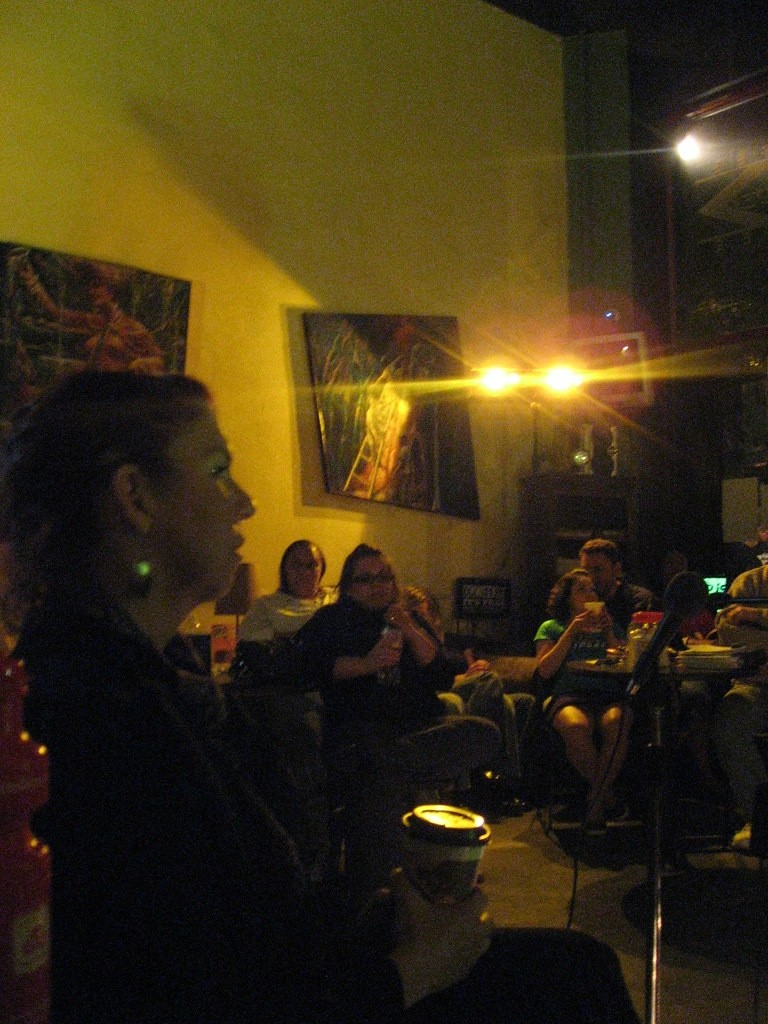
[0,656,54,1024]
[380,623,401,686]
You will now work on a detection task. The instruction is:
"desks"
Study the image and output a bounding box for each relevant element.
[566,660,756,938]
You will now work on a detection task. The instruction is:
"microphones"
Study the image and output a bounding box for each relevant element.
[624,572,709,697]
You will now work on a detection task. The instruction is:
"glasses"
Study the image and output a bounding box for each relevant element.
[347,573,393,586]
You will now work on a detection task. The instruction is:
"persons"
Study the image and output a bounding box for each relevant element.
[0,370,403,1024]
[228,538,768,868]
[11,254,165,376]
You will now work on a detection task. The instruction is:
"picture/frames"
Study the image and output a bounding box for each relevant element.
[456,578,511,620]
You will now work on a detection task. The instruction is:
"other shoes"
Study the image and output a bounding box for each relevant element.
[587,818,608,837]
[733,822,752,850]
[608,801,628,822]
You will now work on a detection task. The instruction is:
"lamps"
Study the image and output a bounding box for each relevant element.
[213,563,263,673]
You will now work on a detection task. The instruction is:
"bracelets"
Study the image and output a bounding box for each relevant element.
[25,274,42,295]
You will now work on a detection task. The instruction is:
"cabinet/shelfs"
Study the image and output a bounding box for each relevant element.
[519,472,642,617]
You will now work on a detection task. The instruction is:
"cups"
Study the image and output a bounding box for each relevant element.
[584,601,605,632]
[400,804,492,906]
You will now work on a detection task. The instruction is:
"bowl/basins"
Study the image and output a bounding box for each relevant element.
[678,654,729,670]
